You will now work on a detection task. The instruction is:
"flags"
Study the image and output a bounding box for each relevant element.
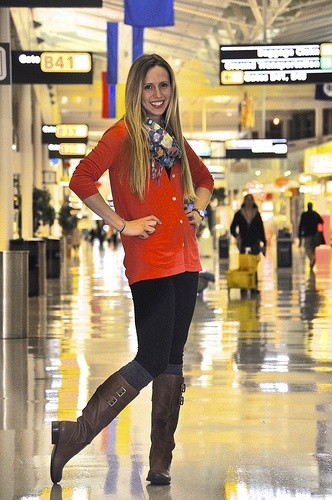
[124,0,174,27]
[107,22,143,83]
[101,71,127,119]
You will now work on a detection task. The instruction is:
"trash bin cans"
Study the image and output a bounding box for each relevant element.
[218,234,230,261]
[0,251,30,339]
[10,239,47,294]
[276,227,294,268]
[42,237,61,278]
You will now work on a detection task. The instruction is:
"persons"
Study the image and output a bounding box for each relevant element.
[230,194,266,294]
[298,203,324,270]
[50,55,215,485]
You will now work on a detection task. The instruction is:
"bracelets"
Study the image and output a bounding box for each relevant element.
[118,219,127,233]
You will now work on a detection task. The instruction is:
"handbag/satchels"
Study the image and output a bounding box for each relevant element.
[317,231,326,246]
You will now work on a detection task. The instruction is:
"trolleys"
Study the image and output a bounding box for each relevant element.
[226,239,261,295]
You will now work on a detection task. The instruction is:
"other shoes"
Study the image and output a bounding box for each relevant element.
[251,289,261,294]
[241,288,246,293]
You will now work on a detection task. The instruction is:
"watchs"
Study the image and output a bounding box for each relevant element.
[192,208,205,220]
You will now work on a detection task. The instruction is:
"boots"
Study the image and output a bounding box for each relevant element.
[145,373,186,484]
[51,371,139,484]
